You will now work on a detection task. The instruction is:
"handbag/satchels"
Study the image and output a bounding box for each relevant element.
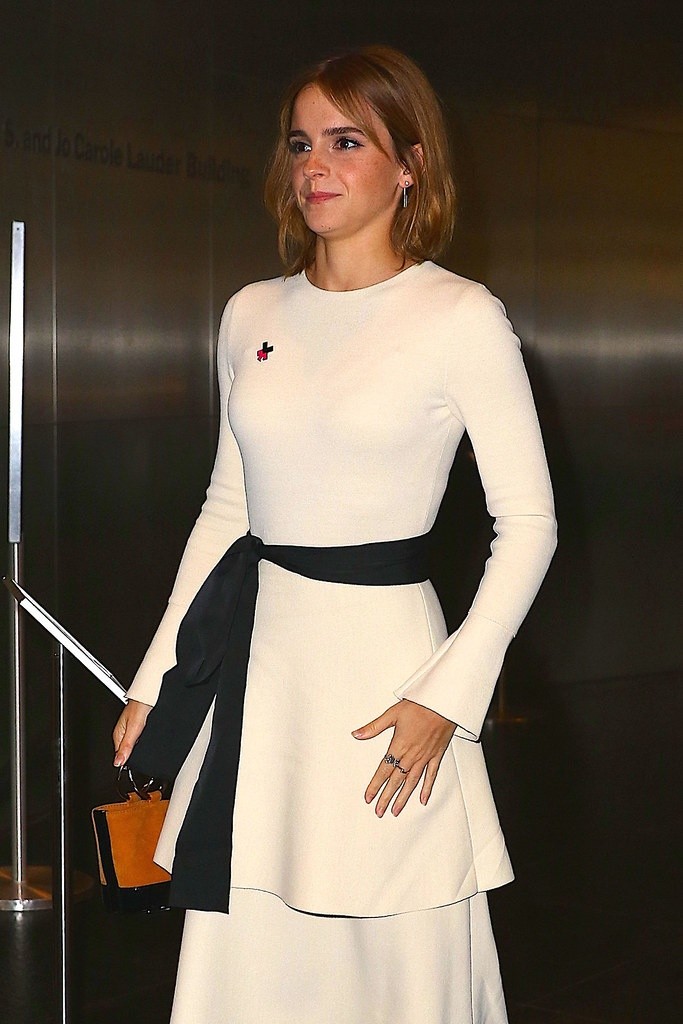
[91,738,182,915]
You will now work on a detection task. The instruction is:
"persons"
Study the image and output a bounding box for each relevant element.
[110,33,560,1022]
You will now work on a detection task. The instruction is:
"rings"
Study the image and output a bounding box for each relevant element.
[384,753,398,768]
[396,765,409,775]
